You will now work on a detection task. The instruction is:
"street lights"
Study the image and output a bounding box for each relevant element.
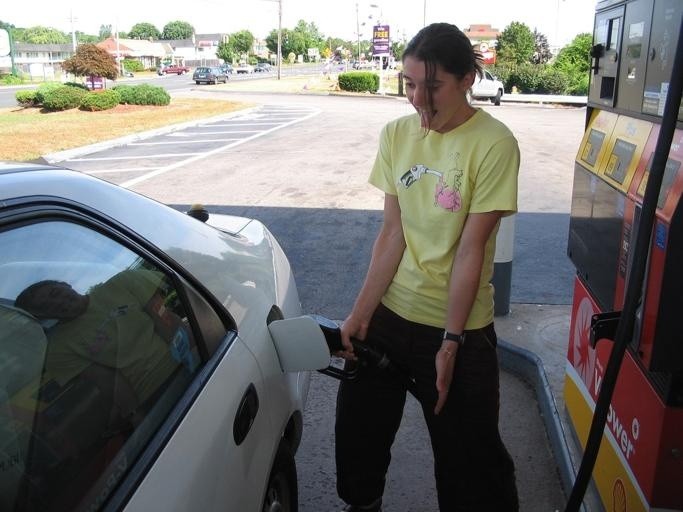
[264,0,281,80]
[355,3,379,62]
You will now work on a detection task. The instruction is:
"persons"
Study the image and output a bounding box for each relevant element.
[12,269,196,430]
[331,25,521,510]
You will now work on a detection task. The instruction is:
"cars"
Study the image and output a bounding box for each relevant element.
[157,64,189,76]
[221,63,271,73]
[193,66,229,85]
[0,163,332,512]
[469,69,504,105]
[353,61,369,70]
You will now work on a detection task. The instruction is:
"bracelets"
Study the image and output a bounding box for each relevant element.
[442,330,466,345]
